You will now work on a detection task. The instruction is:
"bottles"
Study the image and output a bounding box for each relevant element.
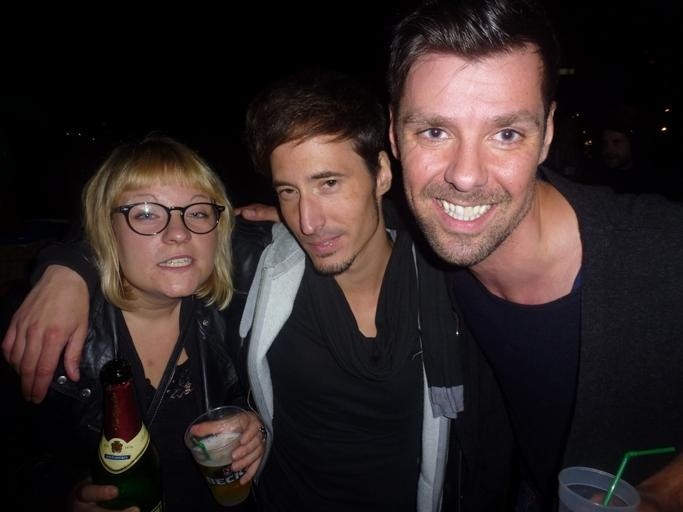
[90,363,167,512]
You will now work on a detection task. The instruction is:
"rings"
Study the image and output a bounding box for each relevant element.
[258,425,267,441]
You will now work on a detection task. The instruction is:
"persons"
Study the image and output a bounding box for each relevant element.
[234,0,682,512]
[16,135,268,512]
[1,88,542,512]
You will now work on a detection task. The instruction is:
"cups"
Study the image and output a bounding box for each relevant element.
[557,465,642,511]
[184,402,254,507]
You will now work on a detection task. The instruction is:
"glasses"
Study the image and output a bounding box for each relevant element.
[113,202,226,234]
